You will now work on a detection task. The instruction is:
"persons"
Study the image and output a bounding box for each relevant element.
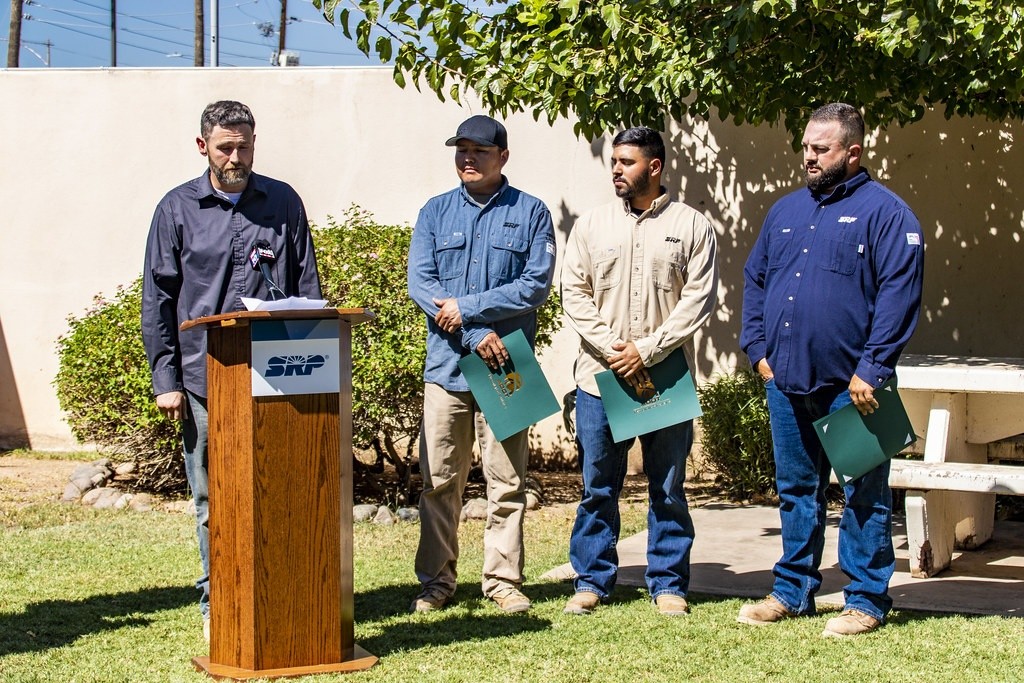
[405,116,557,614]
[142,99,323,627]
[560,128,717,617]
[736,100,922,635]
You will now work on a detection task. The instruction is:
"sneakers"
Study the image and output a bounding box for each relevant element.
[738,595,792,627]
[564,590,602,615]
[648,592,689,619]
[825,606,881,635]
[491,586,531,612]
[411,595,455,614]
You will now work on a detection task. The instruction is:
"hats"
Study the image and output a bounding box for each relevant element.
[446,114,508,146]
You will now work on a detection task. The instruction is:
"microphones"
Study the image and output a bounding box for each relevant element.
[249,238,278,301]
